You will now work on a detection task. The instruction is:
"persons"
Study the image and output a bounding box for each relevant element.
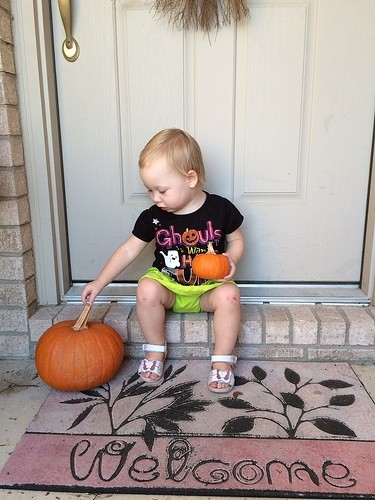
[82,128,245,393]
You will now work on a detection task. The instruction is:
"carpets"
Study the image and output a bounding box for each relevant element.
[0,358,375,500]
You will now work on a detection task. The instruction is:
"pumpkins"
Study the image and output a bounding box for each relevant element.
[191,242,230,279]
[35,303,125,392]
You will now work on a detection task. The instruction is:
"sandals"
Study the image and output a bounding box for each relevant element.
[138,339,167,383]
[208,354,237,392]
